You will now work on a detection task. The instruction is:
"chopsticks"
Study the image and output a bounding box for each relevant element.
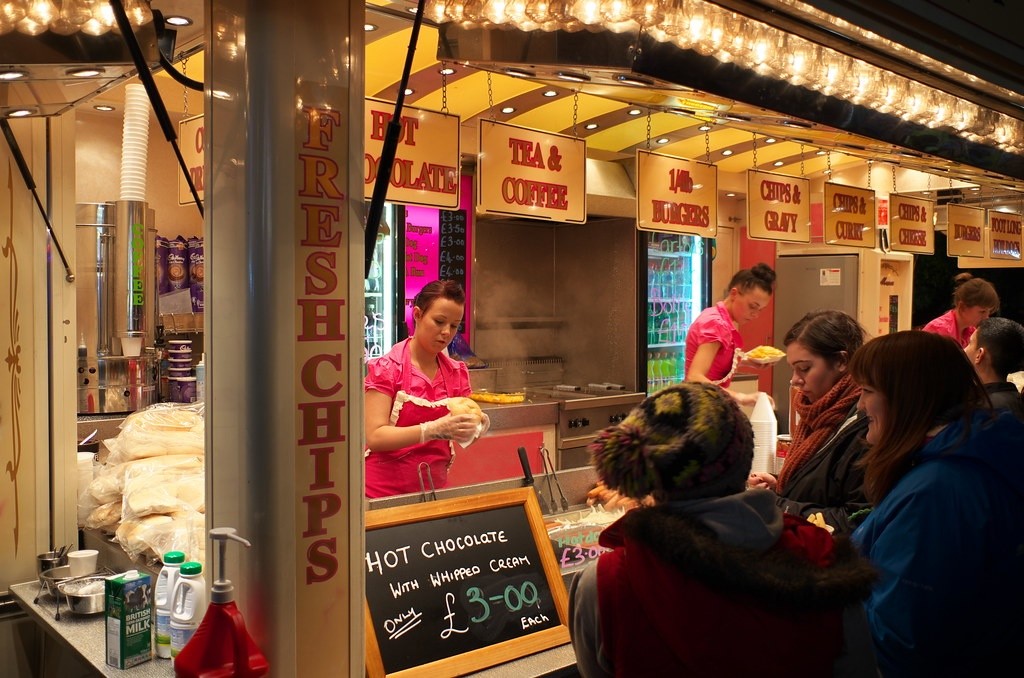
[54,543,73,558]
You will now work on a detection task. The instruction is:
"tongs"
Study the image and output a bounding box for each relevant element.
[540,448,569,515]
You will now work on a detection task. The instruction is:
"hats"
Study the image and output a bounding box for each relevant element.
[587,381,754,507]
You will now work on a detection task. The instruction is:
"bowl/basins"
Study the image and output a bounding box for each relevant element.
[39,564,104,599]
[57,576,105,614]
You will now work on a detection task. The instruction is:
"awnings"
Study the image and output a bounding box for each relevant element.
[0,0,1024,261]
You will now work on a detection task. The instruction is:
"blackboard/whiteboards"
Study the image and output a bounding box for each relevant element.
[364,488,576,678]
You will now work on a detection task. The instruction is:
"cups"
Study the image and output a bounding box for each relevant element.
[121,337,142,356]
[119,83,150,202]
[166,339,197,403]
[38,552,68,588]
[67,549,100,576]
[749,392,778,475]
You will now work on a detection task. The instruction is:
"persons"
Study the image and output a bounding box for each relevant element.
[684,263,776,409]
[922,274,999,349]
[569,309,1024,678]
[364,280,490,498]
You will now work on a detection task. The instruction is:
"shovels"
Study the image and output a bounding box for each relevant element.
[516,446,551,515]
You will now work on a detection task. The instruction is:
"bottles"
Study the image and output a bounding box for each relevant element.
[646,231,696,393]
[195,352,205,403]
[78,451,95,520]
[169,561,206,673]
[154,550,185,659]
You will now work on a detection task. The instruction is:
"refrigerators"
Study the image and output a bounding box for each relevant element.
[555,219,712,400]
